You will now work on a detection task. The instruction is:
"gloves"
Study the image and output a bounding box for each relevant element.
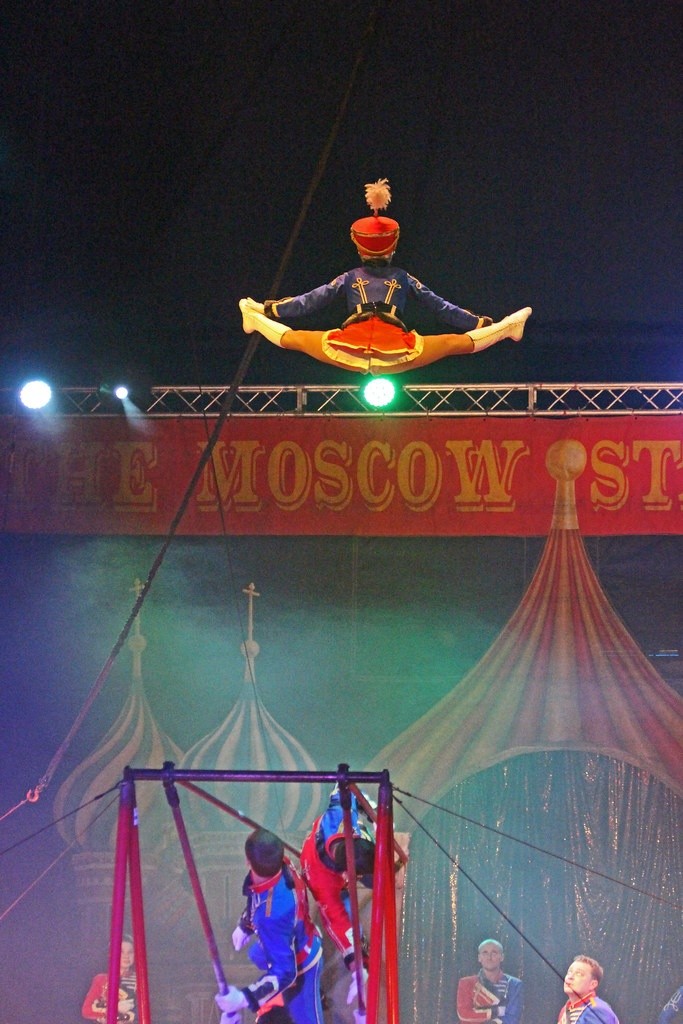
[231,926,250,951]
[117,998,134,1013]
[347,968,369,1005]
[220,1009,241,1024]
[214,987,248,1013]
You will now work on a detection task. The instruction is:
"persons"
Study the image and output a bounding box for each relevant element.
[299,781,377,1005]
[557,954,619,1024]
[456,939,524,1024]
[214,829,324,1024]
[220,1006,293,1024]
[81,936,139,1024]
[658,986,683,1024]
[239,212,533,377]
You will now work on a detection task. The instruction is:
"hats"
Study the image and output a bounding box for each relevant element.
[350,180,401,257]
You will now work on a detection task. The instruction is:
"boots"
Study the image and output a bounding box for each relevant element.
[239,296,290,349]
[468,307,531,354]
